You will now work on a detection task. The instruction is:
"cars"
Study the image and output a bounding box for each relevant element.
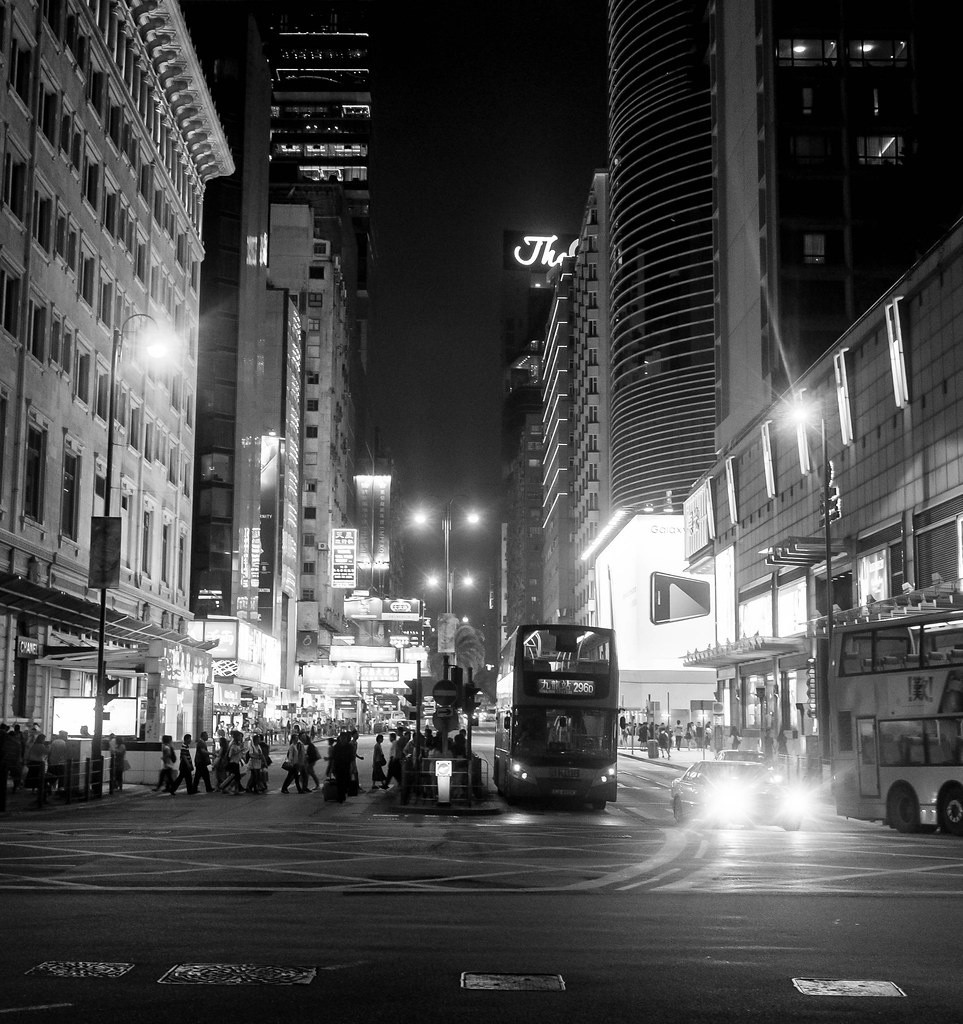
[670,761,802,831]
[715,749,784,785]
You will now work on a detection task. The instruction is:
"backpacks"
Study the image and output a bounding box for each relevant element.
[659,733,669,749]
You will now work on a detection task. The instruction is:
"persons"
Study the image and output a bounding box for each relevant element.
[152,716,470,798]
[0,722,125,795]
[764,729,789,763]
[730,726,742,750]
[674,720,712,752]
[621,722,673,761]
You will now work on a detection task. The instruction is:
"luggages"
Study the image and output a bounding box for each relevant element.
[349,773,359,797]
[648,740,659,758]
[323,777,339,802]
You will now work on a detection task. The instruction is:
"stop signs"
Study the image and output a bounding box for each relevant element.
[433,679,457,707]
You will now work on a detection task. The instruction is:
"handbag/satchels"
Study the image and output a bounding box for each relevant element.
[225,750,232,762]
[281,762,293,773]
[315,747,321,762]
[381,756,386,767]
[683,733,692,739]
[727,735,735,744]
[170,748,176,763]
[123,759,130,772]
[248,757,261,771]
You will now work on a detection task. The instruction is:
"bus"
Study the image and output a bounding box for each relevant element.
[492,623,627,810]
[825,608,963,836]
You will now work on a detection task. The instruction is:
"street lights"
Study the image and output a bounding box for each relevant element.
[414,493,481,680]
[782,399,835,628]
[90,312,177,758]
